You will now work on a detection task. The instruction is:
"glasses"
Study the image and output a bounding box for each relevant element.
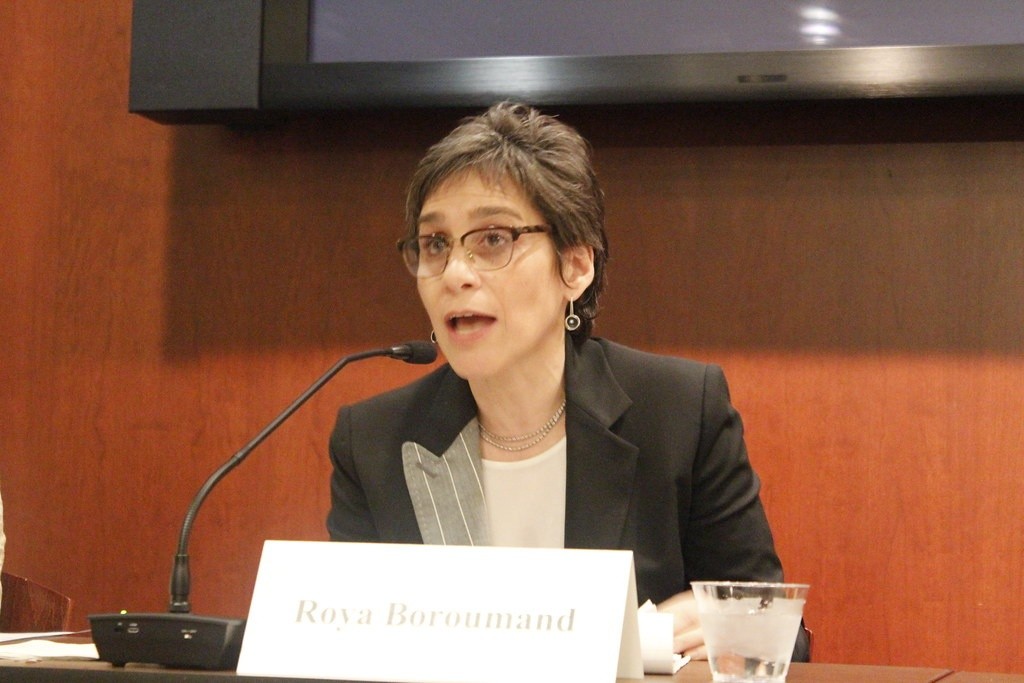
[396,224,553,279]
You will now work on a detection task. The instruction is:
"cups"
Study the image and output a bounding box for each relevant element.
[690,582,811,683]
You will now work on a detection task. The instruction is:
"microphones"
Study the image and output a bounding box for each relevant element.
[86,340,437,670]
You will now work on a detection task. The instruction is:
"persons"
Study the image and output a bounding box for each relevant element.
[328,99,813,663]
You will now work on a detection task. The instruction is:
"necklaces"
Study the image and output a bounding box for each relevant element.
[477,397,566,451]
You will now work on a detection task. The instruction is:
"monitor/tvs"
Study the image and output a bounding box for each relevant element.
[126,0,1023,127]
